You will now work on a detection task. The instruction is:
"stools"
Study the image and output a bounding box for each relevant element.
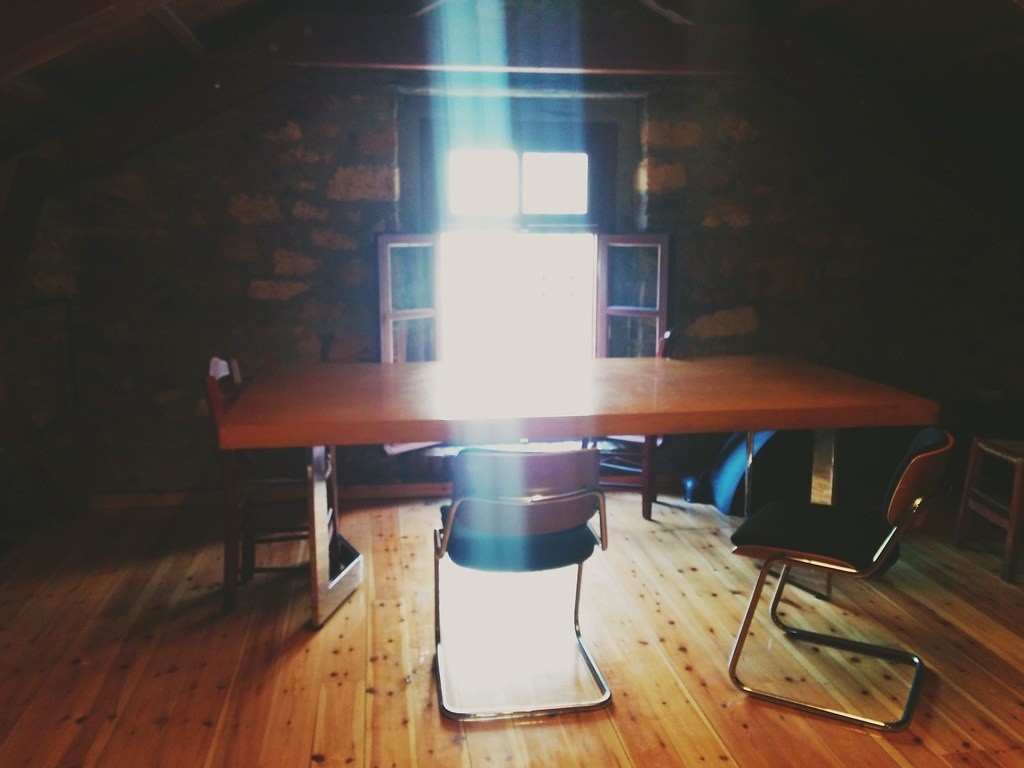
[953,431,1024,584]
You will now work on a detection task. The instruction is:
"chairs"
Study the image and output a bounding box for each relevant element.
[717,421,963,735]
[196,352,341,611]
[426,443,623,728]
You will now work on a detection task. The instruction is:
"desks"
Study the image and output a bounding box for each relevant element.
[212,348,932,642]
